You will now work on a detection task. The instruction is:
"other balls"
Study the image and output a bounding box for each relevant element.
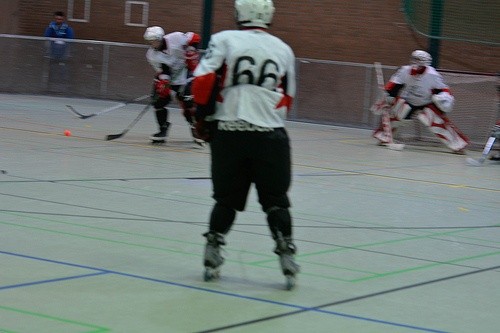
[65,130,72,136]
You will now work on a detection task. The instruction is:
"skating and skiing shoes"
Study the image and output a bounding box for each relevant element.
[202,231,227,281]
[191,127,205,144]
[446,133,468,154]
[373,127,392,144]
[272,239,301,291]
[150,122,170,143]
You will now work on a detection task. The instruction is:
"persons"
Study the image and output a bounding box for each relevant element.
[192,0,301,289]
[371,50,469,154]
[43,11,73,92]
[144,26,207,148]
[488,120,500,161]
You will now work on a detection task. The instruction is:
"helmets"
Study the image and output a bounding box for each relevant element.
[144,26,165,40]
[233,0,275,30]
[411,50,432,66]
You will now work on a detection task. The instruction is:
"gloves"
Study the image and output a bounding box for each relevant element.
[185,50,199,68]
[432,92,454,113]
[156,79,170,97]
[371,100,390,115]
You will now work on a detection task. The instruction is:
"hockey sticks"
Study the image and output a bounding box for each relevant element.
[374,60,406,151]
[105,100,153,140]
[462,128,494,168]
[63,88,170,119]
